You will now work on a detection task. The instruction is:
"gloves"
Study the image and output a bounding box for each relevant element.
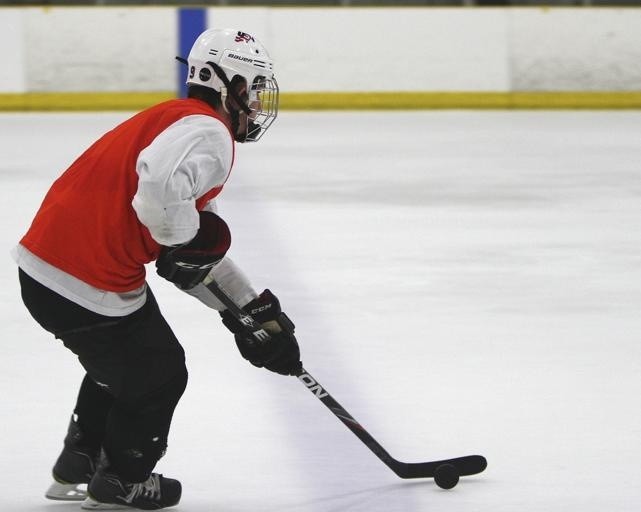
[219,288,300,377]
[155,210,232,292]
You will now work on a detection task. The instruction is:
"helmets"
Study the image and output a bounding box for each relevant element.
[185,27,279,143]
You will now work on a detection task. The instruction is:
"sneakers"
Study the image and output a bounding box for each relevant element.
[52,413,105,486]
[87,456,182,511]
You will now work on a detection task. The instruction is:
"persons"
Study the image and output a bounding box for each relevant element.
[10,29,300,511]
